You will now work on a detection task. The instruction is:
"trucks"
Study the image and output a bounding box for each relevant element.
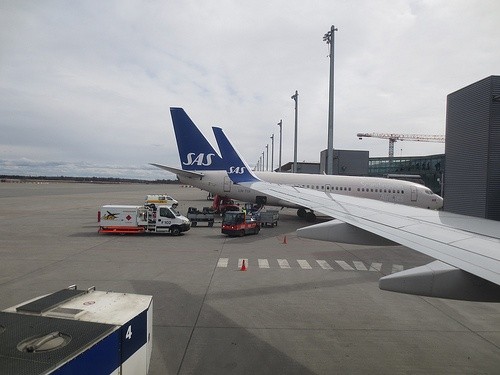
[97,202,192,236]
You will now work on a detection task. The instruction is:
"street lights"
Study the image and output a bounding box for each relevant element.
[277,119,282,172]
[290,90,299,171]
[262,151,265,171]
[265,143,268,171]
[322,25,338,175]
[269,133,275,172]
[254,156,262,171]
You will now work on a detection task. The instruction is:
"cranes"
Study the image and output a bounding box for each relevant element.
[356,132,446,169]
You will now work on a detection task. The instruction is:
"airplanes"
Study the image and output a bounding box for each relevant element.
[148,106,499,285]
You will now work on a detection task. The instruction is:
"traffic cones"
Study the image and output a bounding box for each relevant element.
[282,235,287,244]
[240,259,248,271]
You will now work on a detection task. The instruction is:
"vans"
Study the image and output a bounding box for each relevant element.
[145,195,180,209]
